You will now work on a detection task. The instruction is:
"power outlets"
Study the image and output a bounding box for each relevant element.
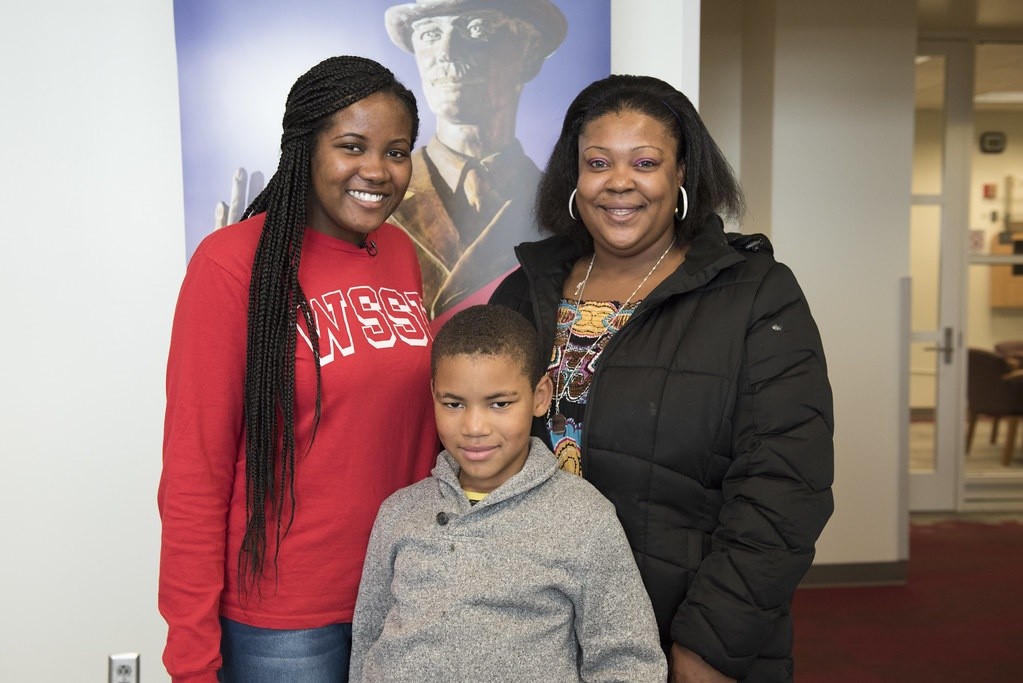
[108,653,140,683]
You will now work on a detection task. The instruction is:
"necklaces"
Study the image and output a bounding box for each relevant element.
[552,234,679,434]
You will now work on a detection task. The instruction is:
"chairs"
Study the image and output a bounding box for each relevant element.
[968,348,1023,466]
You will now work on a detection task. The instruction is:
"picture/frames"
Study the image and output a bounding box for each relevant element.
[982,132,1006,152]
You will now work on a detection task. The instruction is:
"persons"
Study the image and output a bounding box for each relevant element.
[346,303,671,682]
[489,74,836,682]
[158,55,436,681]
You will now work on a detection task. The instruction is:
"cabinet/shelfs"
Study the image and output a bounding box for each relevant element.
[988,231,1023,308]
